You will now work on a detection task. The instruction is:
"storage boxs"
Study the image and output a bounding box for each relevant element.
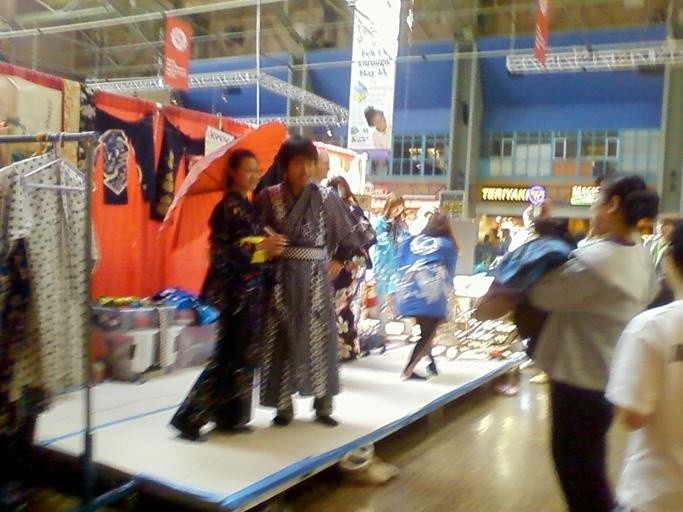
[93,301,222,385]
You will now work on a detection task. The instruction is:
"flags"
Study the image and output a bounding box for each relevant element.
[160,14,193,92]
[532,1,553,67]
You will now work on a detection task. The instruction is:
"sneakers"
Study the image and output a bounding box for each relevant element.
[495,382,520,396]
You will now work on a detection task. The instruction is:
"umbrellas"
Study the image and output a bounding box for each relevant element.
[155,122,289,255]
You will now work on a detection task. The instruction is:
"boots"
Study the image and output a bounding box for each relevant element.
[340,443,399,484]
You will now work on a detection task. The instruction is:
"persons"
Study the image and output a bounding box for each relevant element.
[252,138,354,427]
[511,171,659,512]
[647,211,678,271]
[387,212,458,382]
[600,220,683,511]
[168,147,290,443]
[373,192,411,305]
[327,176,378,363]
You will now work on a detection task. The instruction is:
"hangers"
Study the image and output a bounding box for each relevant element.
[14,132,86,193]
